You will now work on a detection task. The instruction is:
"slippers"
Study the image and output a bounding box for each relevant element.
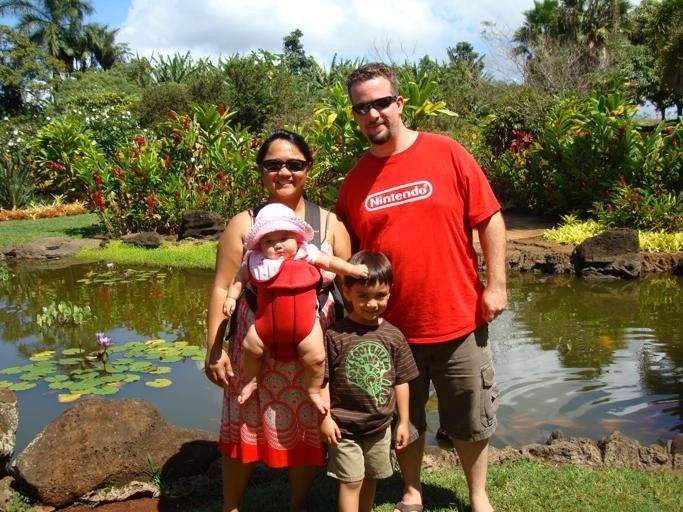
[394,501,426,512]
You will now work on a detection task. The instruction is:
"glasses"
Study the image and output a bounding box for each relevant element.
[257,158,311,172]
[352,95,397,116]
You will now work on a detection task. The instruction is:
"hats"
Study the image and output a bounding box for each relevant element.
[241,203,315,251]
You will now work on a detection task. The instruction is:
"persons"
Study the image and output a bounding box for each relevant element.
[316,250,420,512]
[335,62,509,511]
[219,199,371,417]
[204,129,353,511]
